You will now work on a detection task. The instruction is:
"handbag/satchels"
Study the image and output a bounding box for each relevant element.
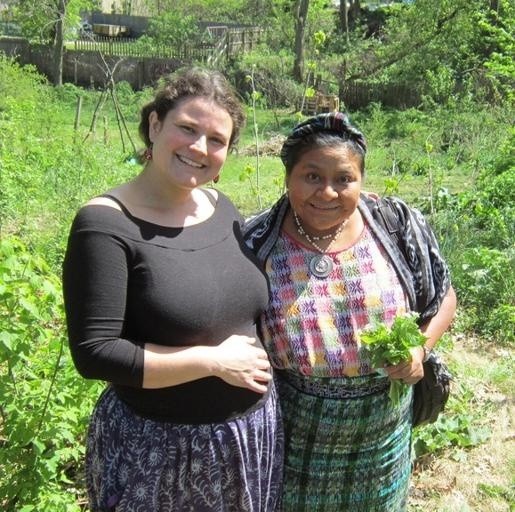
[411,349,451,427]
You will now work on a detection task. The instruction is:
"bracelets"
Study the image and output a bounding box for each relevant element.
[419,343,430,361]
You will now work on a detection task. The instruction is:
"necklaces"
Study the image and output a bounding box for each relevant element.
[293,210,355,279]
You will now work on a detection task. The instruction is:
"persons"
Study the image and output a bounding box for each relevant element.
[62,68,380,510]
[241,112,458,511]
